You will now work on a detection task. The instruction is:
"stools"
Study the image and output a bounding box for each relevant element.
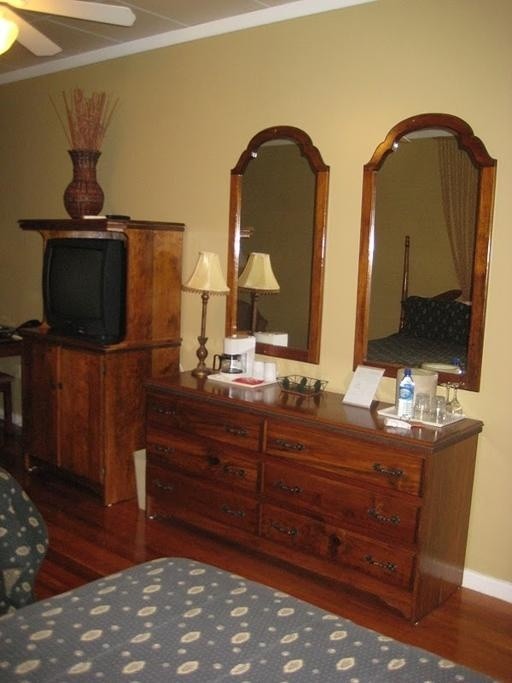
[1,372,15,435]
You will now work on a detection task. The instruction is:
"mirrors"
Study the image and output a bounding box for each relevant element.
[224,127,330,365]
[353,113,497,392]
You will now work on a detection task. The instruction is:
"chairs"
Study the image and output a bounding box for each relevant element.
[0,468,48,620]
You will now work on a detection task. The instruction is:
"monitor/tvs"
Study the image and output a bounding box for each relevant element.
[41,237,126,346]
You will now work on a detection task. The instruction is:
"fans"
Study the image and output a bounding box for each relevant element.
[0,0,136,57]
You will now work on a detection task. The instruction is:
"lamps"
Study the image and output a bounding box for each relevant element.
[237,252,282,335]
[181,250,230,377]
[0,18,18,56]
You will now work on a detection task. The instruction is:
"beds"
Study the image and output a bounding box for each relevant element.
[366,294,470,373]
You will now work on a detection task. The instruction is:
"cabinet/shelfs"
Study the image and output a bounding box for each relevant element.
[145,367,480,622]
[20,219,185,504]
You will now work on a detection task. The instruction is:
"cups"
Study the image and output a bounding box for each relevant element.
[253,361,264,377]
[415,393,428,414]
[430,396,445,418]
[264,363,276,382]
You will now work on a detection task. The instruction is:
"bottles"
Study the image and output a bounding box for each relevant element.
[398,369,415,416]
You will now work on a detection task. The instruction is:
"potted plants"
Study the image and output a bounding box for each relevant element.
[47,86,119,219]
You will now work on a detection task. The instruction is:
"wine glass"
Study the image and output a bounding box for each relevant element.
[448,383,462,416]
[440,381,451,414]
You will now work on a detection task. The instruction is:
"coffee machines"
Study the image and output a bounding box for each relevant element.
[253,331,288,348]
[213,335,255,379]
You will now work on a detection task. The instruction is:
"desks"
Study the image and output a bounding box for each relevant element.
[0,325,21,357]
[0,557,499,681]
